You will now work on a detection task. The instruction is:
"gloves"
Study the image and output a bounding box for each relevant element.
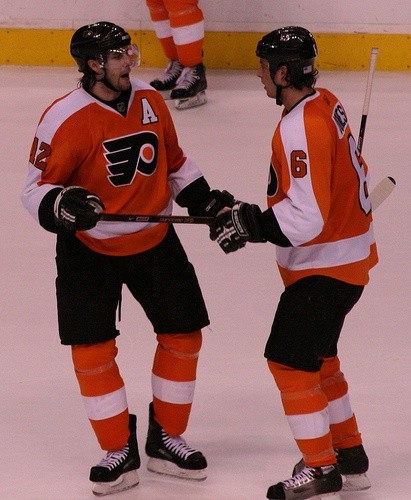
[209,203,261,254]
[54,184,106,231]
[199,188,236,219]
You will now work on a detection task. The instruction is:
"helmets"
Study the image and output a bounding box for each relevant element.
[69,22,133,66]
[256,25,317,65]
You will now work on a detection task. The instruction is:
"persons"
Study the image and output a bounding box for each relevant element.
[20,21,236,496]
[210,25,378,500]
[145,0,208,110]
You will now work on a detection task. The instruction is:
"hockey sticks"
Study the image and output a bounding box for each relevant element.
[94,176,397,223]
[355,48,379,155]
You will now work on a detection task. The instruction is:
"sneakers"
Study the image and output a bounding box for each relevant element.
[292,444,372,492]
[145,402,208,480]
[150,59,184,101]
[89,415,142,496]
[267,449,343,500]
[169,62,207,109]
